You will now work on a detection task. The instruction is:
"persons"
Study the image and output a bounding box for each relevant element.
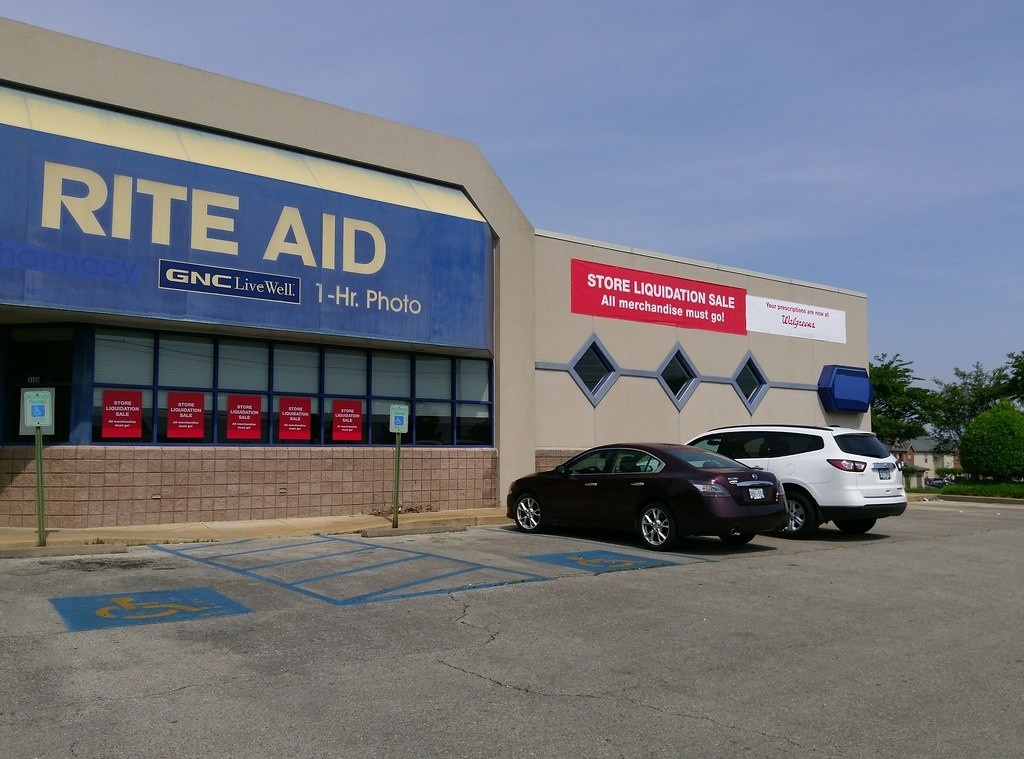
[925,476,930,485]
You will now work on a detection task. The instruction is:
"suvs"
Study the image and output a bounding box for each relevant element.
[635,423,908,540]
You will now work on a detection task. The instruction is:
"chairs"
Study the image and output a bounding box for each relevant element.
[702,462,719,469]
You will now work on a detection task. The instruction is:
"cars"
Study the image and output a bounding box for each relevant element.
[925,478,951,487]
[506,442,787,551]
